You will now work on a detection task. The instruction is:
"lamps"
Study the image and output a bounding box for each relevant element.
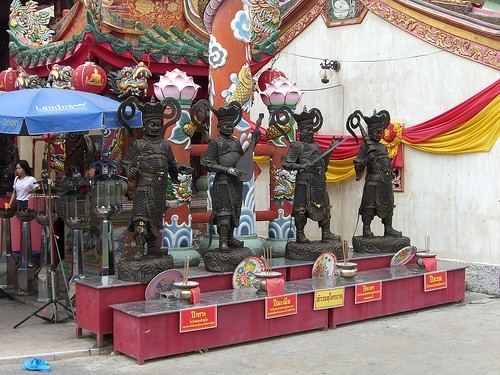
[319,59,340,84]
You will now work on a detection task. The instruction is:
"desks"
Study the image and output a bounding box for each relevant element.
[72,249,470,365]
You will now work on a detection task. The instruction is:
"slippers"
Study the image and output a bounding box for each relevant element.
[24,358,51,372]
[22,357,49,370]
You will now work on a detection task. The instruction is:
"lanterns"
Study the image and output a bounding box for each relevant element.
[72,61,107,94]
[0,69,22,92]
[257,67,287,93]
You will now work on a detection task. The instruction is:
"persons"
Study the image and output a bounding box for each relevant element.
[345,109,405,238]
[6,159,40,270]
[117,96,195,258]
[270,103,342,243]
[189,97,263,253]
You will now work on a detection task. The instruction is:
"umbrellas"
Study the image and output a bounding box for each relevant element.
[0,83,144,267]
